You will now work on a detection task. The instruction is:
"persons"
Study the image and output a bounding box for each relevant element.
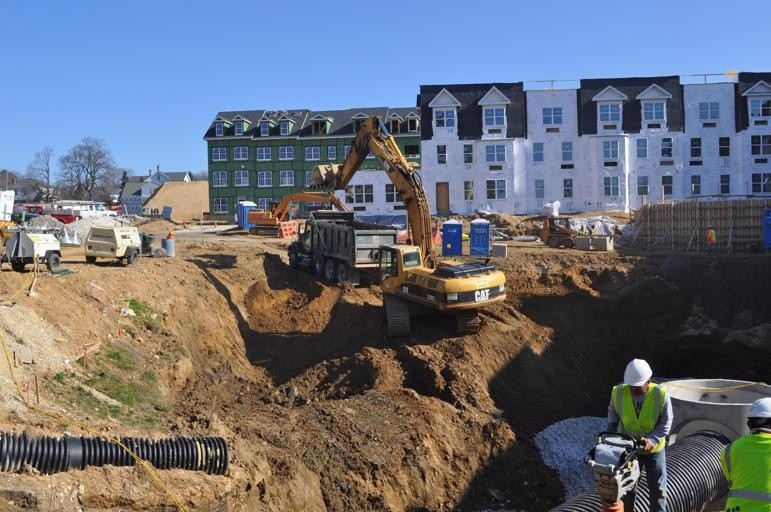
[606,359,674,512]
[704,224,718,253]
[578,225,588,236]
[717,397,771,512]
[588,225,596,236]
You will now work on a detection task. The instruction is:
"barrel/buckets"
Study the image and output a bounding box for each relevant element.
[142,233,154,253]
[161,239,174,257]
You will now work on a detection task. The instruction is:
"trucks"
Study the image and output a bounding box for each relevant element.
[54,199,116,220]
[12,204,75,227]
[286,209,397,287]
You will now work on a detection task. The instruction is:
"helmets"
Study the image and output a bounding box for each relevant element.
[746,398,770,419]
[623,359,652,387]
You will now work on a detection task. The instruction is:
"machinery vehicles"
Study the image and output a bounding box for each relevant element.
[307,112,507,340]
[540,213,578,250]
[245,189,361,239]
